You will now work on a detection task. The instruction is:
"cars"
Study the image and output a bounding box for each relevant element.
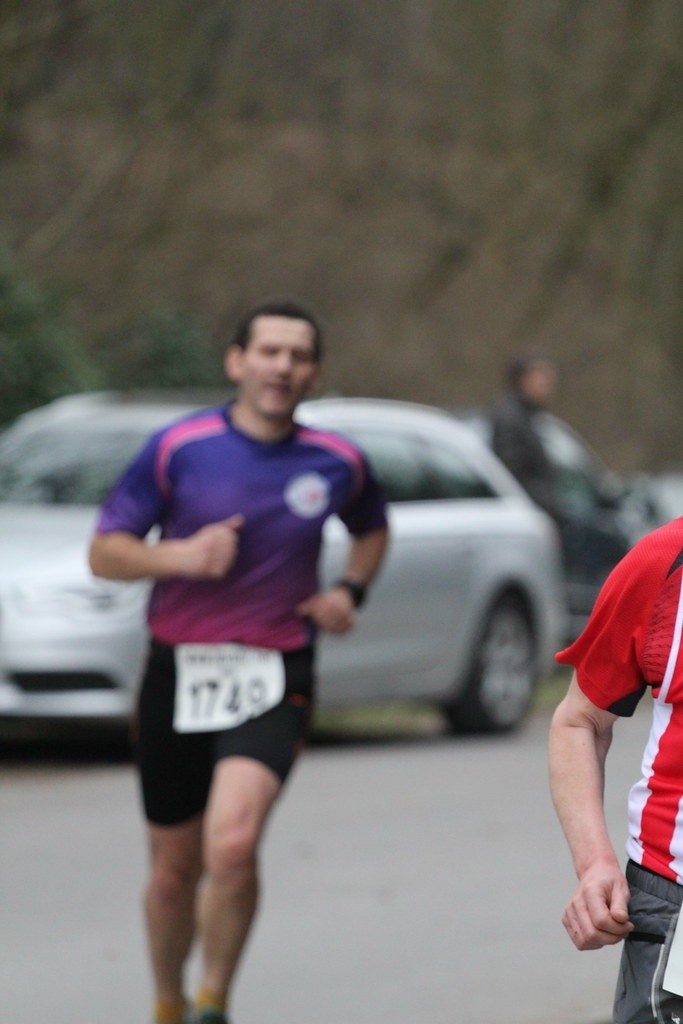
[446,406,661,636]
[0,390,569,739]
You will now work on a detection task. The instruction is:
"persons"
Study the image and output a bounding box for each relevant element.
[88,297,390,1024]
[545,514,682,1024]
[495,352,558,508]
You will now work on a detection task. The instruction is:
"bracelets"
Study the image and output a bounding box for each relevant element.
[333,575,365,609]
